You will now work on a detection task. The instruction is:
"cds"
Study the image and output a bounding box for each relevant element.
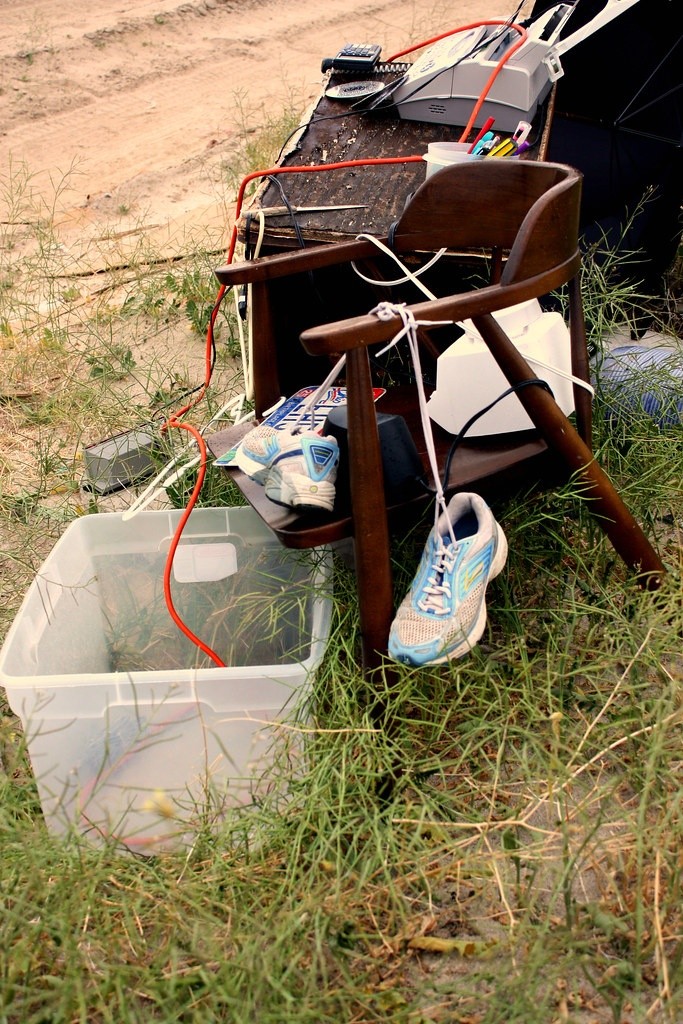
[326,81,385,98]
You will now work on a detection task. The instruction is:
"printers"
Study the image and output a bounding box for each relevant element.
[393,0,640,134]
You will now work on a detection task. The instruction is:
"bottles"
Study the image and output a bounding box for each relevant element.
[584,342,683,427]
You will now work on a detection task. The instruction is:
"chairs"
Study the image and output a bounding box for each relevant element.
[214,155,667,728]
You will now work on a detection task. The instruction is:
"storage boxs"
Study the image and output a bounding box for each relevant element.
[1,505,332,855]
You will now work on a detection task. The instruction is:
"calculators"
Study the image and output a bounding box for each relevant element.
[332,42,382,72]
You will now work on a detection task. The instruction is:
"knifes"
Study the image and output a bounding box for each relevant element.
[242,205,369,216]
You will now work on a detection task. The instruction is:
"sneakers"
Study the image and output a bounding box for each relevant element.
[389,491,509,665]
[236,426,339,512]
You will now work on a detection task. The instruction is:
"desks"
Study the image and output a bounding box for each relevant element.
[236,58,556,395]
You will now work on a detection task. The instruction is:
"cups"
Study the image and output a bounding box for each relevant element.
[426,142,520,180]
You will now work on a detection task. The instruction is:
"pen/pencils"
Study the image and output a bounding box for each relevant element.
[464,115,532,160]
